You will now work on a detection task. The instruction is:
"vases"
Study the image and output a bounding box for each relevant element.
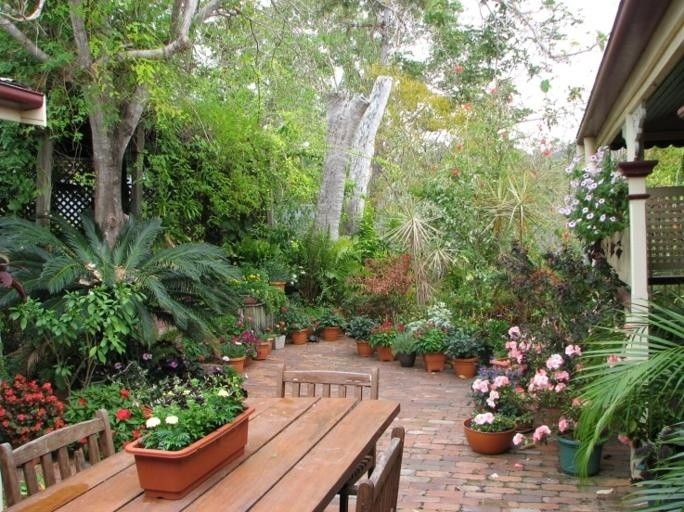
[124,408,255,499]
[558,429,613,478]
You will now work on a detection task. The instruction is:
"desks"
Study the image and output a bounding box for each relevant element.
[0,397,401,512]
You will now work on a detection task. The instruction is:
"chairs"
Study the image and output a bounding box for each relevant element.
[1,408,115,507]
[355,426,405,511]
